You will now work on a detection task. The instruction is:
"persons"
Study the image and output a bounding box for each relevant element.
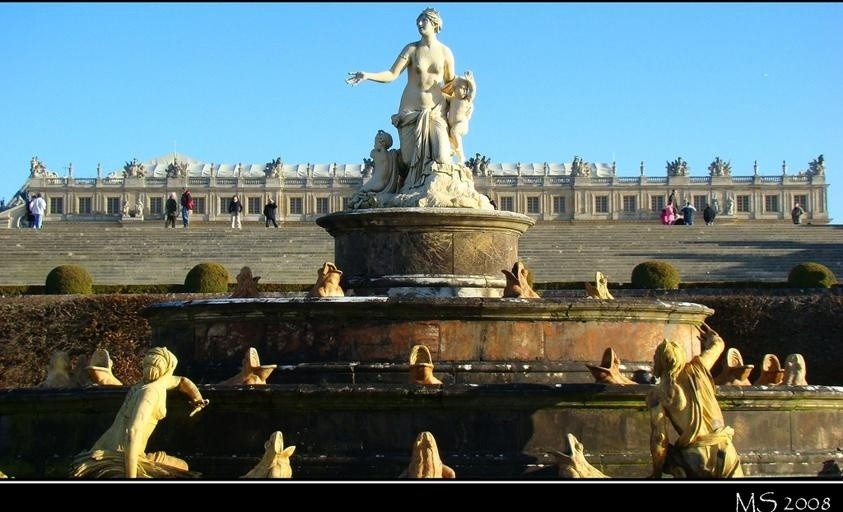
[89,347,210,478]
[164,192,179,228]
[27,193,46,228]
[661,189,697,225]
[645,320,744,478]
[344,6,476,204]
[704,204,716,225]
[181,187,194,228]
[712,195,719,214]
[263,195,278,227]
[228,195,242,228]
[727,196,734,215]
[792,202,804,224]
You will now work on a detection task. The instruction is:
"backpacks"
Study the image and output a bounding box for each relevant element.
[185,191,193,210]
